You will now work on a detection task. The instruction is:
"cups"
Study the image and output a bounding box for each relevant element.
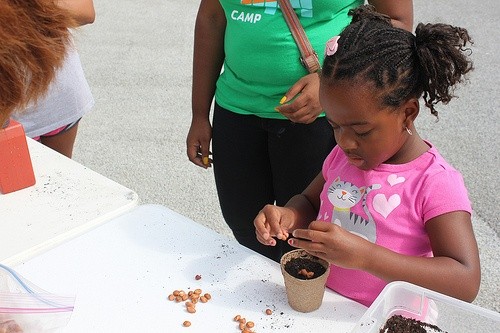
[281,248,330,313]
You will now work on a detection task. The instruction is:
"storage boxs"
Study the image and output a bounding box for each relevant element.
[349,278,500,333]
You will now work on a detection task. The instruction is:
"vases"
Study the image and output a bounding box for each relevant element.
[280,247,331,313]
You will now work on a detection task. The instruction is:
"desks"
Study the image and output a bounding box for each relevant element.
[0,202,373,333]
[0,134,141,275]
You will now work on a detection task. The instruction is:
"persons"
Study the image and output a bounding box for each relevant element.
[253,5,482,325]
[1,0,96,159]
[185,0,414,267]
